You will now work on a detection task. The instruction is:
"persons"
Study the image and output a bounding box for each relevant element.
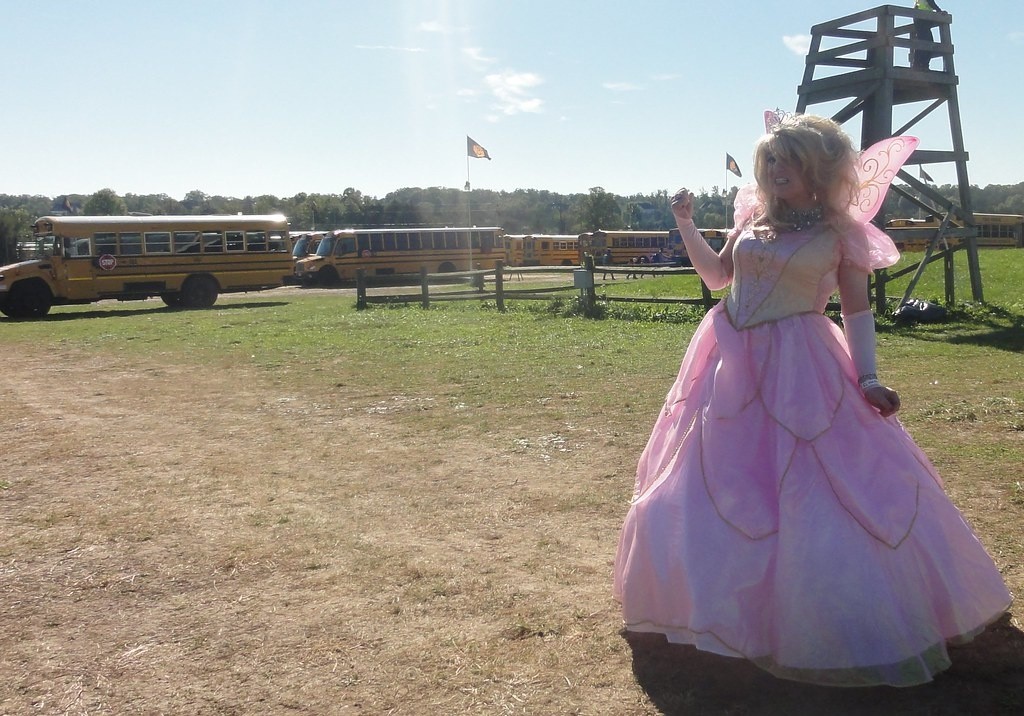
[626,248,666,280]
[603,248,616,281]
[912,0,948,71]
[612,109,1012,688]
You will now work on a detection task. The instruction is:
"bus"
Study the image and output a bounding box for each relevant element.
[523,235,580,266]
[503,234,532,267]
[578,228,734,267]
[0,214,297,321]
[289,227,506,288]
[925,213,1024,250]
[885,218,926,253]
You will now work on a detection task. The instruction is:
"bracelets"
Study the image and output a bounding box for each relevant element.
[856,373,880,389]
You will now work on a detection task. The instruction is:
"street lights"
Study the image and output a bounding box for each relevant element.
[464,182,472,282]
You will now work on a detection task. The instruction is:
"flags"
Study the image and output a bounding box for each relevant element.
[727,155,742,178]
[921,167,933,183]
[467,138,491,161]
